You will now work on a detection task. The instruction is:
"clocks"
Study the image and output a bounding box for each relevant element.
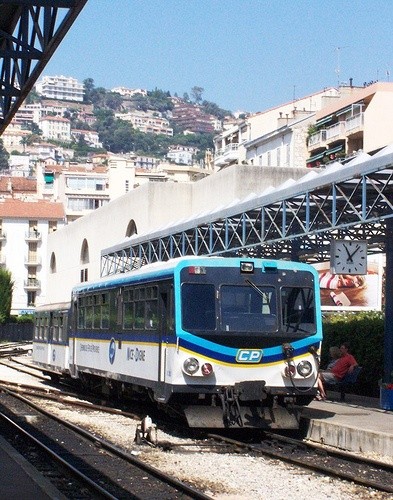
[330,239,367,275]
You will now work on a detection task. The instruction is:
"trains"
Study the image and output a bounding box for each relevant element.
[33,255,324,434]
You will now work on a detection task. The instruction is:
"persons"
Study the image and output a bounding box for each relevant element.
[315,342,359,400]
[318,346,342,372]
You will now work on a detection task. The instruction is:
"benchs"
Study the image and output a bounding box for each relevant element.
[324,366,361,401]
[222,312,279,331]
